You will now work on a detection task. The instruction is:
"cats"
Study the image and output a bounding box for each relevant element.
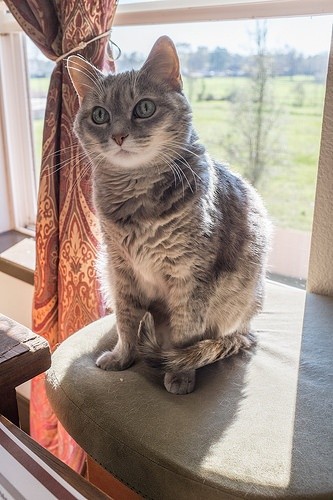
[40,35,273,394]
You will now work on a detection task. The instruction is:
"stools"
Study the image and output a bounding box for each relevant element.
[44,283,333,500]
